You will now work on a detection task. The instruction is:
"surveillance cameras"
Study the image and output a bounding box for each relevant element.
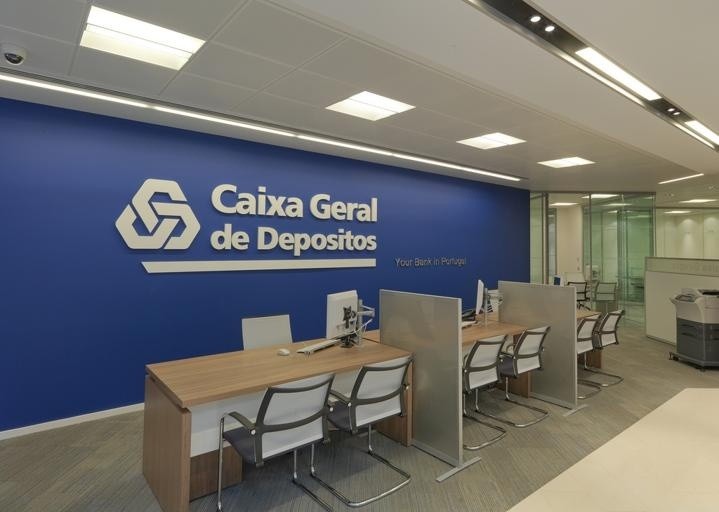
[0,43,28,66]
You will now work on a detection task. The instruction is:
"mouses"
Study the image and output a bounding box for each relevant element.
[276,348,290,356]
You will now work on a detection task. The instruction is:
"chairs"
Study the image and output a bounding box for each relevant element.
[594,281,616,314]
[476,325,549,429]
[460,337,507,450]
[217,374,334,511]
[578,308,625,387]
[313,359,412,505]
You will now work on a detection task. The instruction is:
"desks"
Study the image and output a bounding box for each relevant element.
[363,317,528,447]
[145,333,413,508]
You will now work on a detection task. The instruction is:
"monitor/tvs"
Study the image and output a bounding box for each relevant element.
[475,279,485,315]
[552,275,564,285]
[325,289,358,340]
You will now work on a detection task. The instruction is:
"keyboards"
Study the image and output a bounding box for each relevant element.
[297,339,339,354]
[462,320,477,328]
